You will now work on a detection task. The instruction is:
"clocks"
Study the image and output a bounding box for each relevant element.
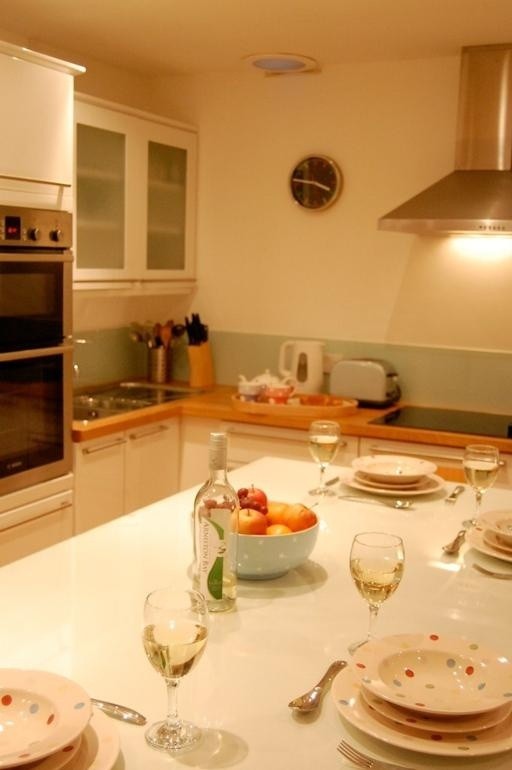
[285,153,345,213]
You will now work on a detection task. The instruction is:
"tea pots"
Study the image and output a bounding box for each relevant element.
[238,368,297,397]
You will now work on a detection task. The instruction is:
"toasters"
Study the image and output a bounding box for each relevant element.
[326,356,402,407]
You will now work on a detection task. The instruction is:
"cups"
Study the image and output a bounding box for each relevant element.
[239,382,267,403]
[268,385,290,405]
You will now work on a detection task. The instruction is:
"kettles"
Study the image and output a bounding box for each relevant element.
[280,336,326,396]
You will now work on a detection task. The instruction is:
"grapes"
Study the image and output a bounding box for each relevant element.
[237,500,248,507]
[236,488,248,496]
[260,505,267,513]
[251,499,261,509]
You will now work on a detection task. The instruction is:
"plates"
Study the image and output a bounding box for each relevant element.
[468,511,512,545]
[453,551,511,579]
[330,665,511,757]
[357,687,511,736]
[334,475,450,500]
[345,472,434,492]
[455,533,510,563]
[60,704,123,770]
[7,731,82,770]
[0,663,93,770]
[351,630,511,716]
[351,453,436,483]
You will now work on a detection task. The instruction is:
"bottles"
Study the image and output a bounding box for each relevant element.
[190,430,241,613]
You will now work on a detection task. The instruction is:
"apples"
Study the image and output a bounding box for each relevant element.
[265,503,291,525]
[285,500,317,528]
[234,507,266,535]
[263,524,291,537]
[237,486,268,508]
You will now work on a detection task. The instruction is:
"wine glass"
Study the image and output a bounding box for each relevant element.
[461,444,501,533]
[345,531,406,660]
[305,419,342,499]
[140,588,210,751]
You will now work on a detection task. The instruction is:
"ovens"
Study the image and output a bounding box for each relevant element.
[0,172,91,501]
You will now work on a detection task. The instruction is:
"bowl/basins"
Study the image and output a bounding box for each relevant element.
[223,509,319,582]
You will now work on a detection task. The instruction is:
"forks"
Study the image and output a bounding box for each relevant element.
[445,486,464,505]
[334,740,422,770]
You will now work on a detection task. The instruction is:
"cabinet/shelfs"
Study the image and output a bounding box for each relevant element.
[74,92,200,294]
[73,406,181,531]
[224,422,505,485]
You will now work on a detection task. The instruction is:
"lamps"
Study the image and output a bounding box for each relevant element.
[244,55,316,76]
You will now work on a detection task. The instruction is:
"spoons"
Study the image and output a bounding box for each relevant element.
[287,656,346,719]
[314,490,413,510]
[443,530,466,553]
[124,318,185,350]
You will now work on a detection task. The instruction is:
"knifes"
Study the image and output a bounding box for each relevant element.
[182,309,215,391]
